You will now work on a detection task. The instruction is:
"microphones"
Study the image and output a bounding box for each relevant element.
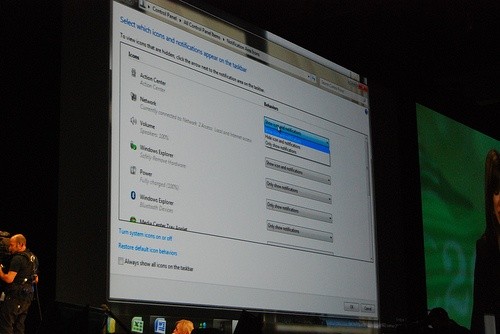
[100,304,131,334]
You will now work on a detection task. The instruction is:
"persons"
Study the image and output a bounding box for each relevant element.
[0,233,38,334]
[172,320,194,334]
[471,148,500,334]
